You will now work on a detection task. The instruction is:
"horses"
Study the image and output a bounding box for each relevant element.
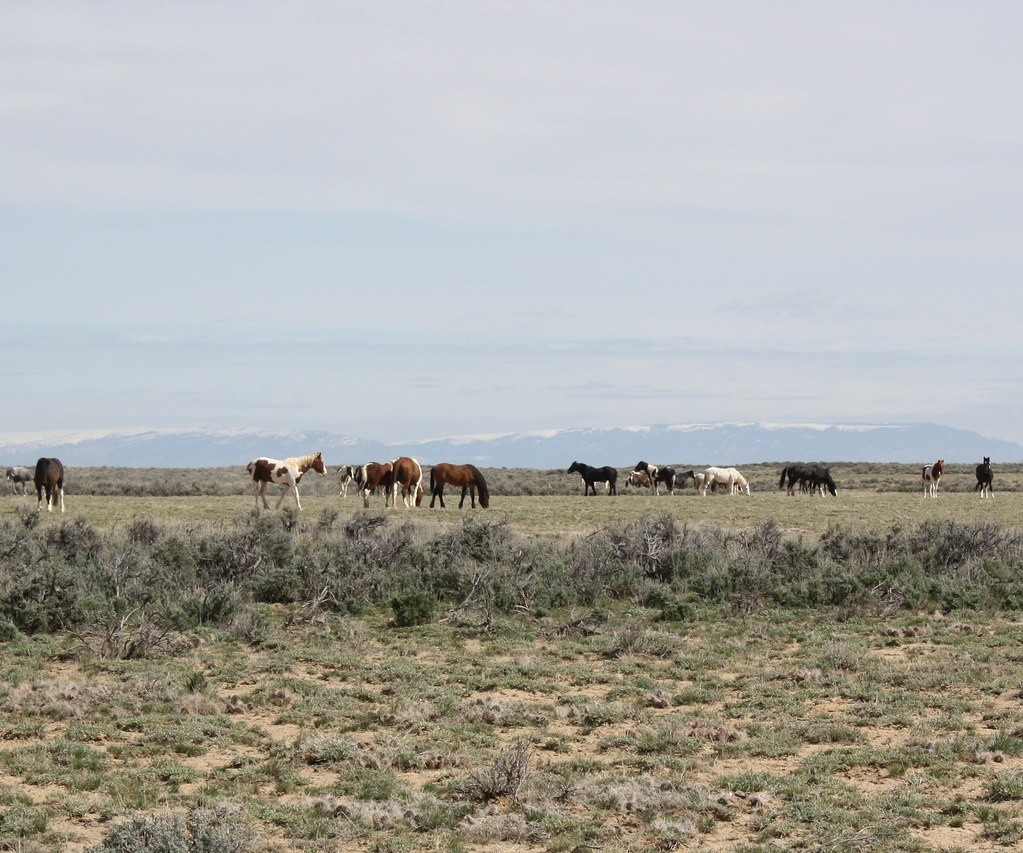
[922,460,944,497]
[336,465,354,496]
[246,452,328,510]
[6,467,46,497]
[34,457,65,513]
[779,463,838,497]
[974,457,994,498]
[429,463,491,509]
[354,457,424,508]
[568,461,620,497]
[625,461,751,496]
